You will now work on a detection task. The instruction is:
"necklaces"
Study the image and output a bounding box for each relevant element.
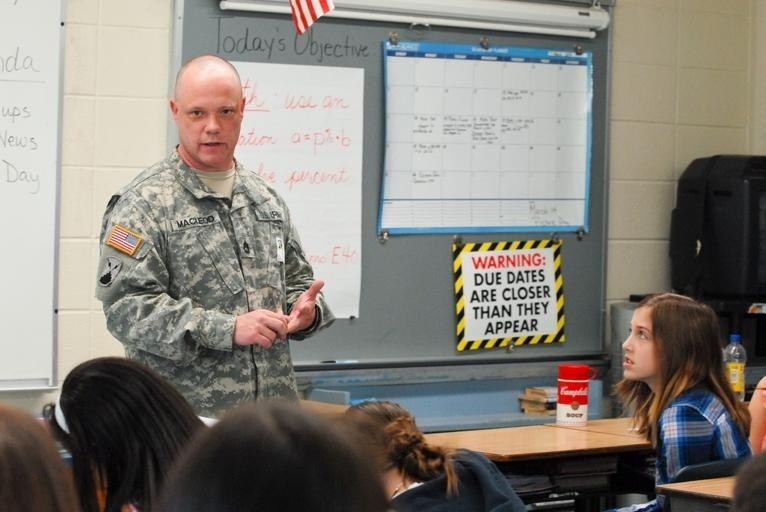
[392,481,409,497]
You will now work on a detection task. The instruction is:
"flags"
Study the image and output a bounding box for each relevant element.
[289,0,335,36]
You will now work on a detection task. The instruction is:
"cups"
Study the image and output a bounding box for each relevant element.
[557,362,596,428]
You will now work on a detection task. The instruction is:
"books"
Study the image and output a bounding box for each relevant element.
[519,386,557,416]
[620,450,656,477]
[547,454,618,490]
[494,462,559,496]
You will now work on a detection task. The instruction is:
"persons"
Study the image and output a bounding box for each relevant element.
[155,397,389,511]
[43,357,211,511]
[610,292,755,511]
[733,451,765,510]
[746,374,766,454]
[1,405,79,511]
[93,54,334,422]
[333,400,526,511]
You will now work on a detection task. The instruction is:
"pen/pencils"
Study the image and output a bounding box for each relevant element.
[322,360,358,364]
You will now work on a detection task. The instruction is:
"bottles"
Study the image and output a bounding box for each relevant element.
[724,334,748,402]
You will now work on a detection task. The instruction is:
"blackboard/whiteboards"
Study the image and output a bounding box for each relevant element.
[0,0,67,392]
[166,0,614,373]
